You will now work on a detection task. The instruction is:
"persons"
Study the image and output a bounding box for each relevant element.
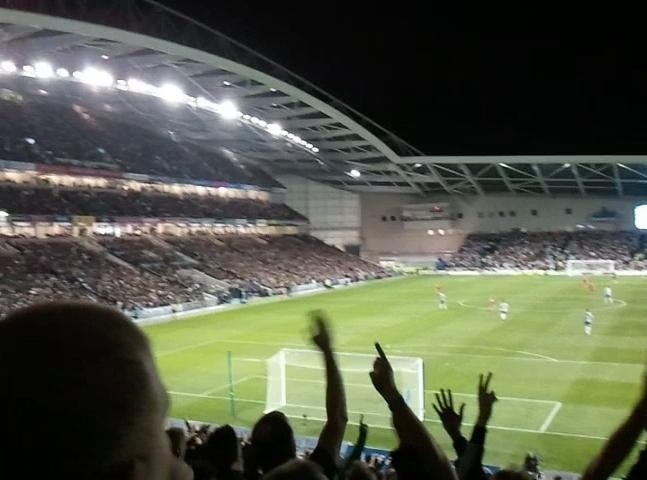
[433,227,647,332]
[0,95,395,315]
[1,300,647,480]
